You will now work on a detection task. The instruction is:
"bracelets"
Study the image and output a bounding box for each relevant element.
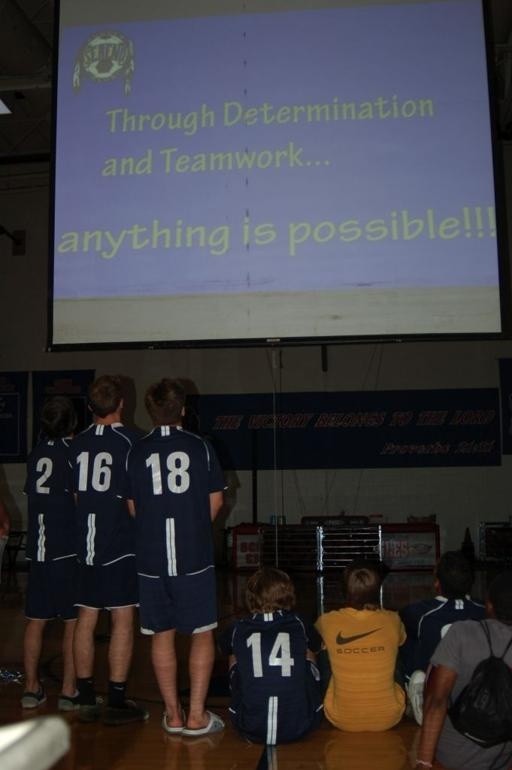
[415,758,435,768]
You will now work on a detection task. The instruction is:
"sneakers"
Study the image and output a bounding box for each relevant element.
[78,697,103,723]
[21,685,48,709]
[57,689,81,711]
[407,669,426,726]
[103,699,149,725]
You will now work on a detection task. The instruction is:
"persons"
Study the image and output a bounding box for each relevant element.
[407,561,511,769]
[396,553,485,720]
[71,375,150,726]
[126,377,226,737]
[226,564,322,744]
[313,559,408,733]
[1,498,50,687]
[19,370,105,713]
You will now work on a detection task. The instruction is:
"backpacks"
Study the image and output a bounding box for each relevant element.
[445,619,512,748]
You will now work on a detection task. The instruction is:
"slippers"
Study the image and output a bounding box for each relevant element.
[182,710,226,736]
[161,708,187,734]
